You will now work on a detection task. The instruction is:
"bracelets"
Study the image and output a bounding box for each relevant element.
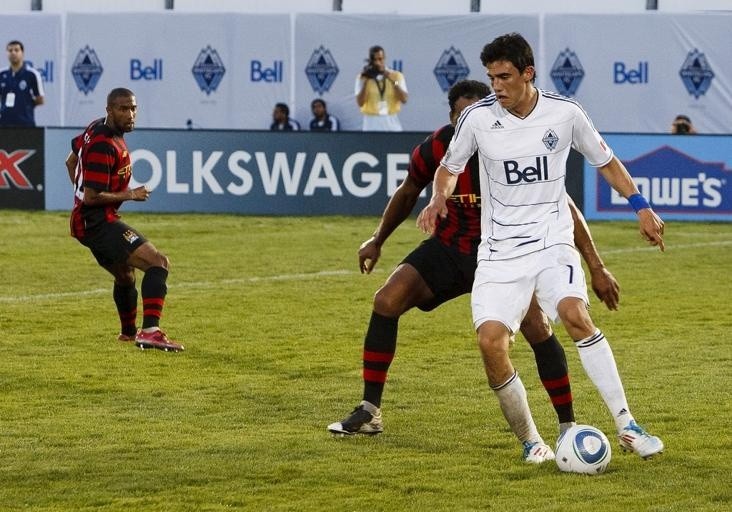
[627,193,651,214]
[393,80,398,87]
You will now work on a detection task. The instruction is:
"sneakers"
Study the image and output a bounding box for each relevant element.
[118,328,145,341]
[521,442,555,465]
[327,403,385,437]
[135,328,186,353]
[619,429,664,462]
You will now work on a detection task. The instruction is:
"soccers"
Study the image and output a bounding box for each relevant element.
[555,425,612,476]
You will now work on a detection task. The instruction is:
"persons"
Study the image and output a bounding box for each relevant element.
[308,98,340,132]
[327,77,619,439]
[354,45,409,131]
[269,102,301,130]
[413,26,666,464]
[671,114,698,134]
[0,40,44,128]
[65,87,186,353]
